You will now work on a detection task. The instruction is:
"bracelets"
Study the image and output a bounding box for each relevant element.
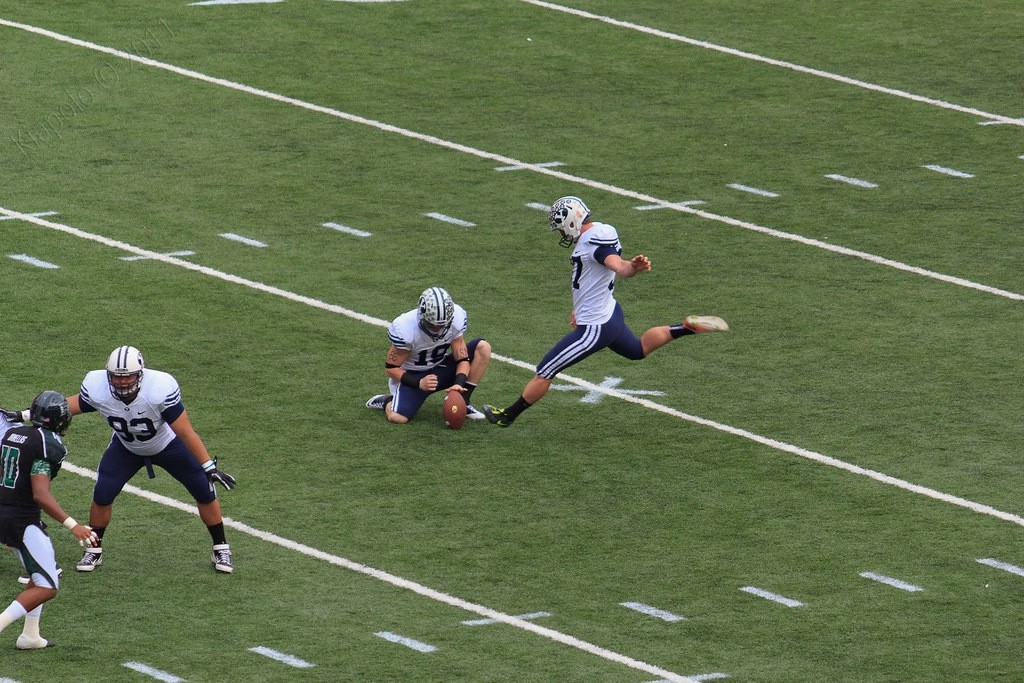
[201,460,216,472]
[63,516,78,531]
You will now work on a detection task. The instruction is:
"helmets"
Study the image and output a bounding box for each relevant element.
[105,345,145,402]
[549,195,591,248]
[30,390,73,438]
[417,286,455,342]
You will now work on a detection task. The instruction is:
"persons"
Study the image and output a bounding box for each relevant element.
[365,287,490,423]
[481,197,730,429]
[0,390,101,648]
[0,407,63,584]
[0,345,236,573]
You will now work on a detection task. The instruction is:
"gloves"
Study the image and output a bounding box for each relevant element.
[201,455,236,493]
[0,405,32,422]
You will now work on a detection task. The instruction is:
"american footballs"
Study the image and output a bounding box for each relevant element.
[442,390,467,430]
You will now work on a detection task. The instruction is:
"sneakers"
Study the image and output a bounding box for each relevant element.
[482,404,514,428]
[75,547,104,572]
[15,634,55,650]
[365,393,394,411]
[17,560,63,584]
[683,313,729,333]
[210,544,233,573]
[465,404,486,420]
